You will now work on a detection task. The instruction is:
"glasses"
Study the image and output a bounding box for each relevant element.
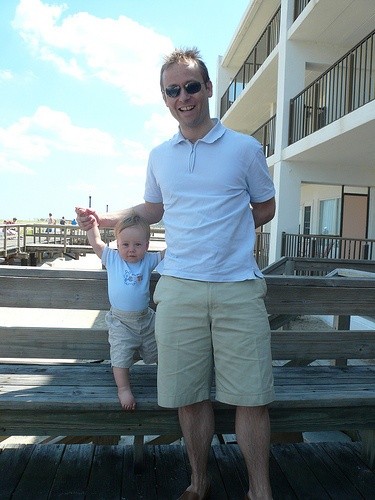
[163,80,202,98]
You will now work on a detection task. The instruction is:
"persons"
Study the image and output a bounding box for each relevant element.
[72,218,76,225]
[74,48,276,500]
[60,216,65,231]
[2,218,17,240]
[44,213,53,241]
[75,207,167,410]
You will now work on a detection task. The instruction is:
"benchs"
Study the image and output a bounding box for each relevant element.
[0,265,375,470]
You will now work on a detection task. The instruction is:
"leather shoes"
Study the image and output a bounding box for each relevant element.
[176,478,210,500]
